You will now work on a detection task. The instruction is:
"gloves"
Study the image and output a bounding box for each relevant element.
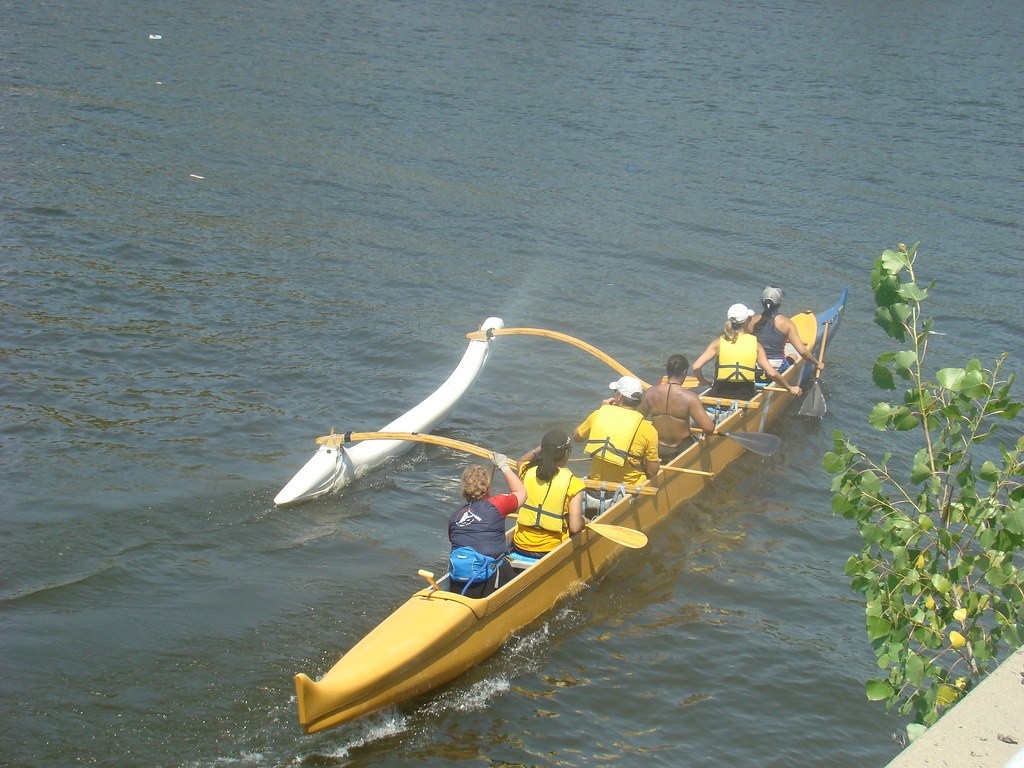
[488,452,511,473]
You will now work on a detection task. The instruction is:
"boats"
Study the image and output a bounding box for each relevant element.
[273,287,852,737]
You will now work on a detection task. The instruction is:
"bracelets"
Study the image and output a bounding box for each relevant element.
[532,448,537,455]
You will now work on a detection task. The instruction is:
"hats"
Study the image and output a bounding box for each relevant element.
[542,430,573,453]
[727,303,755,323]
[762,287,785,307]
[609,376,642,400]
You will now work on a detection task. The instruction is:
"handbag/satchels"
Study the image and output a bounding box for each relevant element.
[448,546,504,583]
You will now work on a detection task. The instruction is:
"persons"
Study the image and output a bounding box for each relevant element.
[572,375,661,500]
[512,429,590,559]
[747,285,826,383]
[634,352,715,459]
[692,303,803,410]
[446,452,528,599]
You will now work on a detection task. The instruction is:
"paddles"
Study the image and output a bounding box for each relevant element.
[689,426,782,459]
[798,319,834,418]
[566,457,716,481]
[662,374,789,395]
[507,513,649,551]
[418,567,439,593]
